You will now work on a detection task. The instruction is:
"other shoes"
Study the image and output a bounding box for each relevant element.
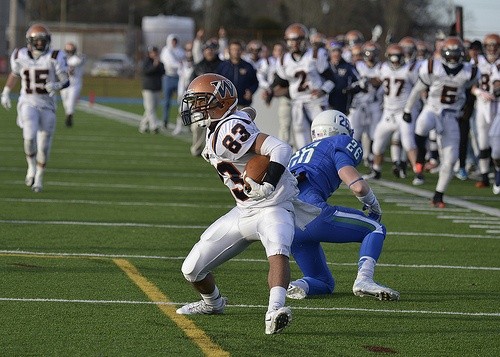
[65,115,72,127]
[432,199,447,209]
[412,149,427,187]
[25,166,35,186]
[424,158,500,195]
[31,172,44,194]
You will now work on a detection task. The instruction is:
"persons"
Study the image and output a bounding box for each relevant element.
[176,74,300,334]
[137,23,500,208]
[288,110,401,301]
[1,25,70,192]
[58,41,84,127]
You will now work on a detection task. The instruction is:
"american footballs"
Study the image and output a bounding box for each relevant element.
[243,155,270,189]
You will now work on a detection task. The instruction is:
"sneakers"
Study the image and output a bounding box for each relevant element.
[361,171,380,180]
[265,306,292,335]
[286,284,307,299]
[175,296,226,314]
[392,165,407,179]
[352,279,401,301]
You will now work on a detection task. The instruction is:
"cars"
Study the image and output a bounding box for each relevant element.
[91,54,136,80]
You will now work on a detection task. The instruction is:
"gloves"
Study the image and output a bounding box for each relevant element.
[0,87,11,111]
[45,82,62,97]
[363,195,383,222]
[243,177,275,201]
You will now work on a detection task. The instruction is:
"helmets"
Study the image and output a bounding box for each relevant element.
[285,24,310,54]
[64,43,75,56]
[180,73,237,127]
[310,109,351,141]
[24,24,50,55]
[310,30,500,69]
